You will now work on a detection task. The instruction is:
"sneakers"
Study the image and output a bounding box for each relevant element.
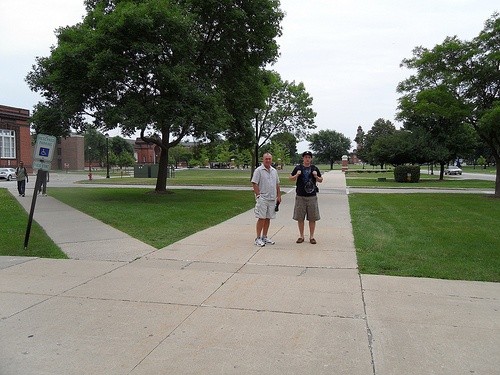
[254,238,265,246]
[262,236,275,245]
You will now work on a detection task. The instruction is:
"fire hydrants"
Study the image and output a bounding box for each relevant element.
[88,173,93,180]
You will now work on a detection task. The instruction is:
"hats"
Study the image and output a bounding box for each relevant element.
[302,151,312,158]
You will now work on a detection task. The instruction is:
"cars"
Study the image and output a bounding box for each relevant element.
[444,165,463,176]
[0,167,17,181]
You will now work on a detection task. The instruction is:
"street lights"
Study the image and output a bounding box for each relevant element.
[254,107,259,169]
[105,133,110,178]
[88,146,92,172]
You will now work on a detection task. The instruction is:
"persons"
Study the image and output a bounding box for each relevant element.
[38,170,49,196]
[64,161,69,174]
[290,151,323,244]
[251,153,281,247]
[15,161,28,197]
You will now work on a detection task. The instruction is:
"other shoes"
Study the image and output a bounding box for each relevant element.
[310,238,316,244]
[296,237,304,243]
[22,194,24,197]
